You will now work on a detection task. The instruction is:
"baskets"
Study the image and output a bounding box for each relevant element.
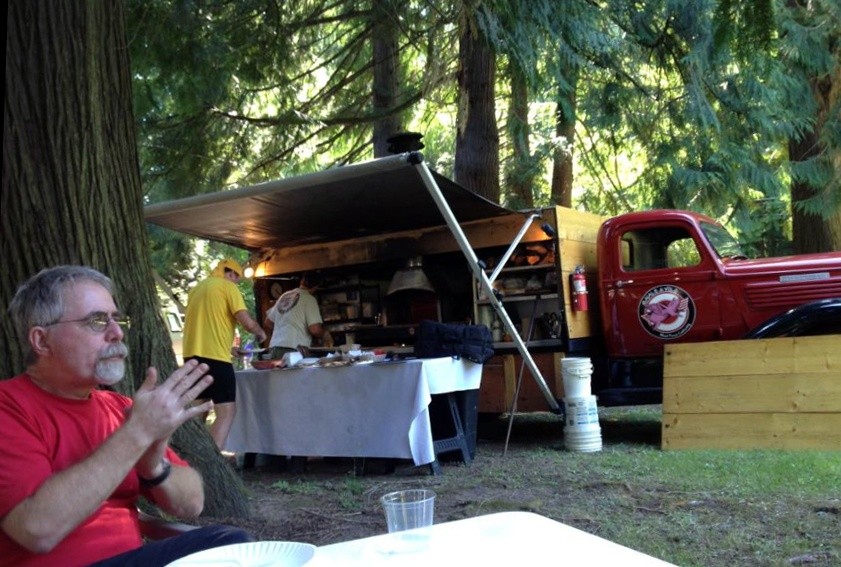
[251,360,281,369]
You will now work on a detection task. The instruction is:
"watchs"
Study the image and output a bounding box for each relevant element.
[135,456,171,488]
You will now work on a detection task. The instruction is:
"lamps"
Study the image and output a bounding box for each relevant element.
[541,223,557,238]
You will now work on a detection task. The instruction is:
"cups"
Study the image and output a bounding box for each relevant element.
[346,301,387,326]
[380,489,438,534]
[345,331,355,345]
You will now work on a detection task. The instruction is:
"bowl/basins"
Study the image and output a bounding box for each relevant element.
[281,352,303,367]
[364,352,388,363]
[251,359,282,369]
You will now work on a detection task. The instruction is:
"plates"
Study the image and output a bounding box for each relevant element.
[296,357,374,368]
[162,541,317,567]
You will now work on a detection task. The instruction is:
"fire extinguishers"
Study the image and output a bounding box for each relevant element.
[570,265,588,311]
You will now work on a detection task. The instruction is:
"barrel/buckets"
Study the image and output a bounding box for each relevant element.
[560,358,602,454]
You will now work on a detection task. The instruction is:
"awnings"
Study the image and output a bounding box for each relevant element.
[143,152,531,253]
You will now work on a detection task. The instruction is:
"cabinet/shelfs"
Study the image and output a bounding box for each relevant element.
[317,282,386,324]
[475,263,558,344]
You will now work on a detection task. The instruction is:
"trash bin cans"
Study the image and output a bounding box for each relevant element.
[380,489,437,534]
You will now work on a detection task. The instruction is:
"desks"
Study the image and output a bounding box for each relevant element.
[223,356,486,475]
[314,511,676,567]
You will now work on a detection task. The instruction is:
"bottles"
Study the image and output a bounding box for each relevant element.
[232,328,255,371]
[491,311,561,342]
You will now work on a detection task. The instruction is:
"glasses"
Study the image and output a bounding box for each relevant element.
[46,312,131,331]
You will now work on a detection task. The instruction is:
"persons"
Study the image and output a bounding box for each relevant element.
[182,258,267,451]
[263,270,334,360]
[0,265,260,567]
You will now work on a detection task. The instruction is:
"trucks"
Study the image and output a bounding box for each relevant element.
[144,149,840,411]
